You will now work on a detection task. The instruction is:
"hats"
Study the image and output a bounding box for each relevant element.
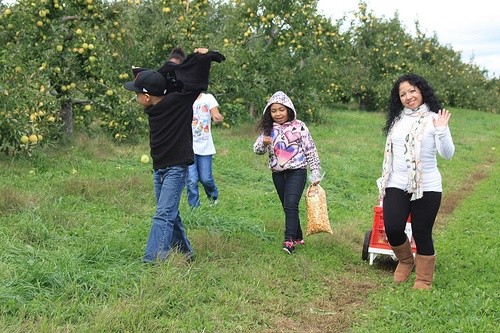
[124,69,168,96]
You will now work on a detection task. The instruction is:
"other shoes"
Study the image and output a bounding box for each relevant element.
[209,198,219,207]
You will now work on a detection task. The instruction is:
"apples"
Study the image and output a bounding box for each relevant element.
[260,0,487,110]
[0,0,257,174]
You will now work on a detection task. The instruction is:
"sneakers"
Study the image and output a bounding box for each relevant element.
[282,240,295,255]
[293,239,305,245]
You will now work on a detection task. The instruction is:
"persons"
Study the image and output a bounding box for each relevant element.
[378,73,455,291]
[183,92,224,206]
[253,91,327,255]
[123,48,209,264]
[155,49,185,71]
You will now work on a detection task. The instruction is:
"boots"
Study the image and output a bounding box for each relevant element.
[388,233,415,284]
[412,251,435,290]
